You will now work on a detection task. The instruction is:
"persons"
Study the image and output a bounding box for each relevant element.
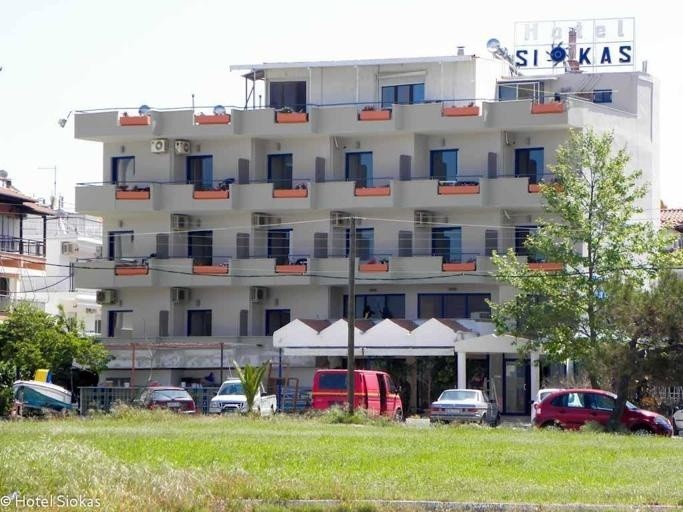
[14,400,22,415]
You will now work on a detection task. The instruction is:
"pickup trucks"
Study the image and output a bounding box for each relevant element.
[208,377,277,418]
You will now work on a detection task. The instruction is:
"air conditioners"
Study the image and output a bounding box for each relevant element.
[171,214,189,230]
[255,214,272,226]
[253,288,267,301]
[150,139,168,152]
[329,212,351,227]
[416,211,434,227]
[172,287,191,303]
[174,139,192,155]
[96,289,115,303]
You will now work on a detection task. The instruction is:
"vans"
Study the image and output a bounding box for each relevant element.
[311,369,404,422]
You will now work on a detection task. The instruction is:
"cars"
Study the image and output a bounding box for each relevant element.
[530,388,583,422]
[430,388,502,428]
[671,405,683,437]
[131,386,196,416]
[533,389,673,438]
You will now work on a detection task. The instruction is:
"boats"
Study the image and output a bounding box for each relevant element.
[12,369,72,414]
